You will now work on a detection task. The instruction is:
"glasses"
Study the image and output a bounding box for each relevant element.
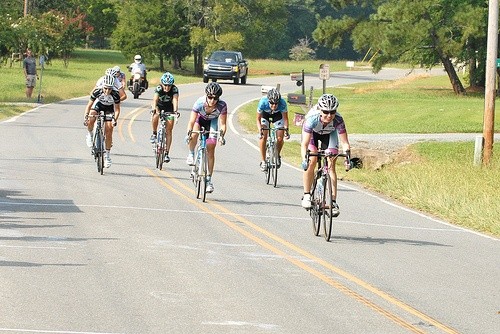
[269,102,278,105]
[104,87,111,90]
[323,110,337,114]
[164,85,172,86]
[208,96,218,100]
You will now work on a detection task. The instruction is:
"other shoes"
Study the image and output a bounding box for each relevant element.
[332,202,341,216]
[164,154,172,163]
[261,161,268,172]
[302,194,314,209]
[187,152,196,165]
[150,135,157,143]
[272,157,282,168]
[206,179,215,192]
[86,133,94,147]
[104,155,114,168]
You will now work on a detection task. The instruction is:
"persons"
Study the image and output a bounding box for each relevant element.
[127,55,146,84]
[300,94,352,217]
[187,82,228,193]
[256,88,290,171]
[150,72,179,163]
[84,66,127,169]
[23,49,40,101]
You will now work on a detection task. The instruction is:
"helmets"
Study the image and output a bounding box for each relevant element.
[267,89,282,102]
[134,55,143,64]
[103,75,114,88]
[160,72,175,84]
[317,94,339,111]
[104,68,119,77]
[205,82,223,95]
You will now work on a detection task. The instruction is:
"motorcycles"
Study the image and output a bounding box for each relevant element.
[126,63,150,99]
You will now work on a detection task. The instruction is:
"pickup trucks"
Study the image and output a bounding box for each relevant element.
[203,51,248,84]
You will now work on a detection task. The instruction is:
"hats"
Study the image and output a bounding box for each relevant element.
[112,66,121,71]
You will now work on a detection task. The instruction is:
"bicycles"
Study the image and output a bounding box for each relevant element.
[85,108,116,175]
[304,147,346,242]
[188,126,224,202]
[260,118,289,187]
[150,110,180,169]
